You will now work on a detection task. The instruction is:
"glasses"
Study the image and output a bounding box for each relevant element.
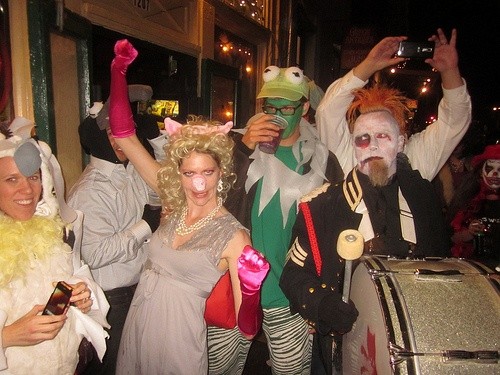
[261,104,301,116]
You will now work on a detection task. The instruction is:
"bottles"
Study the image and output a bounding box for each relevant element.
[474,219,486,259]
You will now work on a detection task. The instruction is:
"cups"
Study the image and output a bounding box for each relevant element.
[258,114,288,154]
[147,186,161,211]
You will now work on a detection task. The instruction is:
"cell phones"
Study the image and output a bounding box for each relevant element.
[398,41,435,58]
[42,281,73,315]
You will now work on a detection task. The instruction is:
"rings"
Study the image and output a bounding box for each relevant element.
[441,42,448,45]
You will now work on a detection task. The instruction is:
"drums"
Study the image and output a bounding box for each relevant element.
[331,255,500,375]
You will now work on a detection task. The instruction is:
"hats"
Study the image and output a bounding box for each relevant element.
[471,144,500,168]
[256,66,310,101]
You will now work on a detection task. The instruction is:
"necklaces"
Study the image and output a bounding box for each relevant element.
[175,196,222,237]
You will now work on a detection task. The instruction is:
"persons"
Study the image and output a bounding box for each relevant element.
[0,116,93,375]
[66,103,161,375]
[112,41,271,375]
[314,28,500,375]
[279,84,455,375]
[206,65,343,375]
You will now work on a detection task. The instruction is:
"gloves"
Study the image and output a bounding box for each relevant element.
[143,204,161,233]
[319,291,360,333]
[238,245,270,335]
[109,39,139,137]
